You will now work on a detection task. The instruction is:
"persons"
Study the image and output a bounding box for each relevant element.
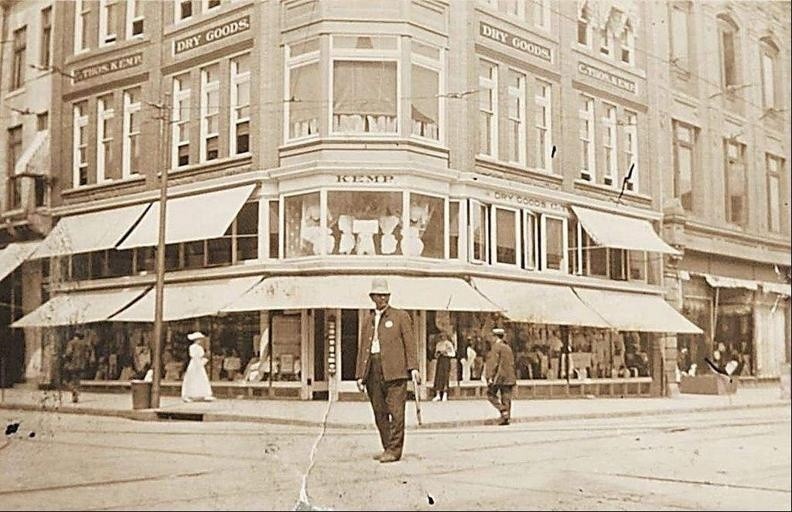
[181,332,216,403]
[613,341,691,377]
[485,329,516,426]
[62,331,88,401]
[432,332,456,402]
[355,277,421,462]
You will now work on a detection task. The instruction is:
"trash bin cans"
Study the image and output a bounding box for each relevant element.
[131,379,153,409]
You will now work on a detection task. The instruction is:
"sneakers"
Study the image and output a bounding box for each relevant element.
[499,414,511,425]
[373,452,400,463]
[432,396,448,401]
[182,397,216,402]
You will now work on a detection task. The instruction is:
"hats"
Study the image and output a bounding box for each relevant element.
[187,331,206,341]
[369,278,391,296]
[492,328,506,336]
